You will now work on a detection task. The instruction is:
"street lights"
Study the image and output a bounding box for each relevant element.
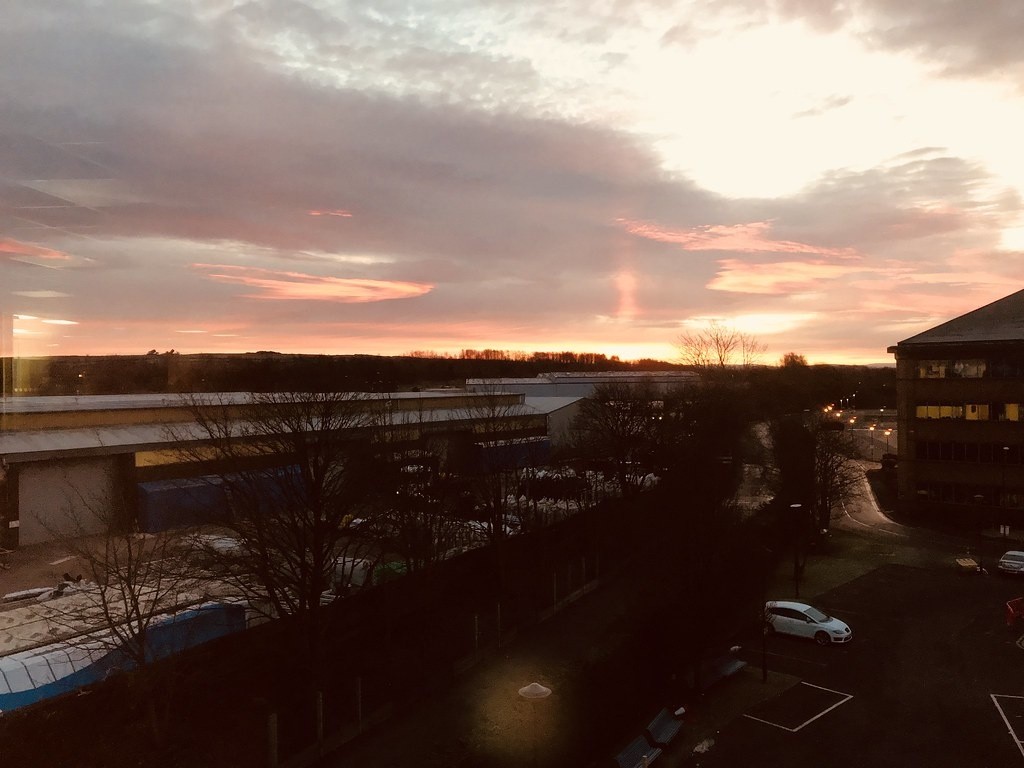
[883,431,890,454]
[837,414,841,421]
[850,419,855,438]
[869,427,874,444]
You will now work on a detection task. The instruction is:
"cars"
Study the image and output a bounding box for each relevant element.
[999,551,1024,575]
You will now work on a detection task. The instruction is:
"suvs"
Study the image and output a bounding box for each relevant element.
[761,601,853,645]
